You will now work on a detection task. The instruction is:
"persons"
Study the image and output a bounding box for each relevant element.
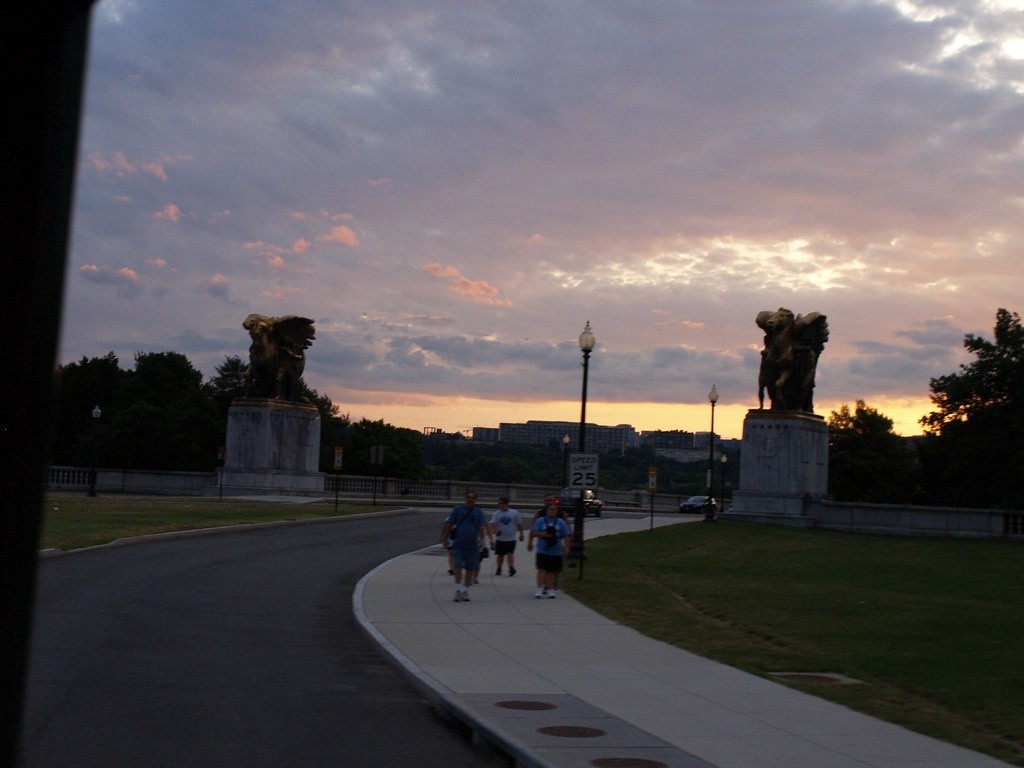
[442,490,494,602]
[488,497,524,576]
[440,504,486,585]
[527,494,571,598]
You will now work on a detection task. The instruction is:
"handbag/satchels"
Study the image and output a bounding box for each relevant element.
[448,528,458,550]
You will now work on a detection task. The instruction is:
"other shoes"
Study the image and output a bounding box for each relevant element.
[496,569,502,575]
[543,588,547,593]
[449,570,455,575]
[548,591,556,598]
[454,589,461,602]
[475,577,479,584]
[509,568,515,575]
[536,590,543,596]
[462,590,471,600]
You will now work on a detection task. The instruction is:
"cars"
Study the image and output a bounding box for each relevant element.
[553,486,603,518]
[680,496,716,514]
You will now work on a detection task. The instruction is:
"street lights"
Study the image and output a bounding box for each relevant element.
[559,434,571,490]
[87,405,103,497]
[717,454,728,512]
[704,383,719,523]
[568,320,596,581]
